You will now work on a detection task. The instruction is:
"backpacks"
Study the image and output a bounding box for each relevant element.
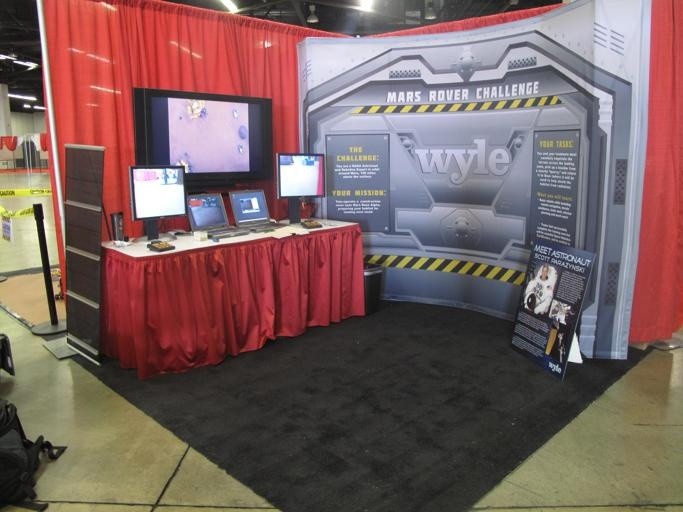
[0,396,44,509]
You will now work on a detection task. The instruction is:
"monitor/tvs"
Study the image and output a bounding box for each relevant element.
[134,88,272,189]
[278,153,326,198]
[131,166,187,220]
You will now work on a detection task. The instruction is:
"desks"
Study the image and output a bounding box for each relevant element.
[102,218,367,378]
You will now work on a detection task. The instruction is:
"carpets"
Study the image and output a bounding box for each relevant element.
[73,298,656,512]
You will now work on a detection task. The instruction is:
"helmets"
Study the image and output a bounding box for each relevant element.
[523,292,536,312]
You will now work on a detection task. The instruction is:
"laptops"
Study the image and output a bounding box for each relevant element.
[229,190,291,232]
[186,193,250,240]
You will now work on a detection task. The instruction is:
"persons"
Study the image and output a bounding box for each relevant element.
[522,264,554,315]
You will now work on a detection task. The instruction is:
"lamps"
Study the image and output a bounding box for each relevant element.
[424,2,437,19]
[307,5,319,22]
